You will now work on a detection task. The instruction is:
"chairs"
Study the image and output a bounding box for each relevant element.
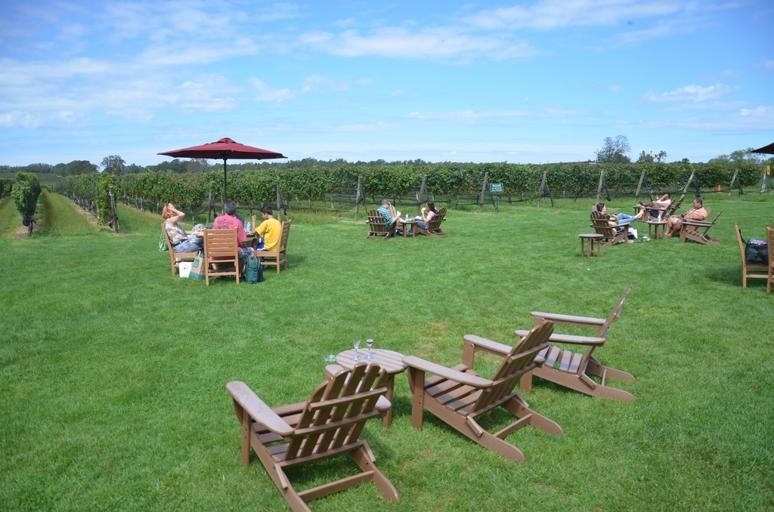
[226,362,400,512]
[680,208,726,245]
[203,226,239,287]
[667,207,713,237]
[514,285,636,402]
[365,210,396,240]
[734,224,774,289]
[590,211,629,248]
[402,319,563,465]
[633,198,675,222]
[414,207,447,238]
[255,219,292,274]
[161,223,198,276]
[766,226,774,294]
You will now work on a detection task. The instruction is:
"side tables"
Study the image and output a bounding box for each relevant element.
[335,348,409,429]
[645,220,669,240]
[400,221,418,238]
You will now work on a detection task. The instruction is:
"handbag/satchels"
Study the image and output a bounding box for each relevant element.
[189,256,205,281]
[745,238,768,264]
[159,231,168,251]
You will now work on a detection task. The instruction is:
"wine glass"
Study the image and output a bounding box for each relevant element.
[365,337,374,353]
[352,339,361,357]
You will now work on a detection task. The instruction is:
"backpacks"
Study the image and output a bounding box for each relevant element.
[245,259,263,282]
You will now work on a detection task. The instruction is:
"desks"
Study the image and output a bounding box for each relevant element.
[578,234,604,259]
[194,231,258,247]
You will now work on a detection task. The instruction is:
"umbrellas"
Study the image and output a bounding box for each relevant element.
[157,137,288,204]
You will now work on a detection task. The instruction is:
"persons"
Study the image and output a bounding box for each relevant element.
[376,199,438,236]
[212,202,251,259]
[664,197,708,237]
[161,202,204,253]
[639,192,672,218]
[597,202,645,233]
[251,206,284,251]
